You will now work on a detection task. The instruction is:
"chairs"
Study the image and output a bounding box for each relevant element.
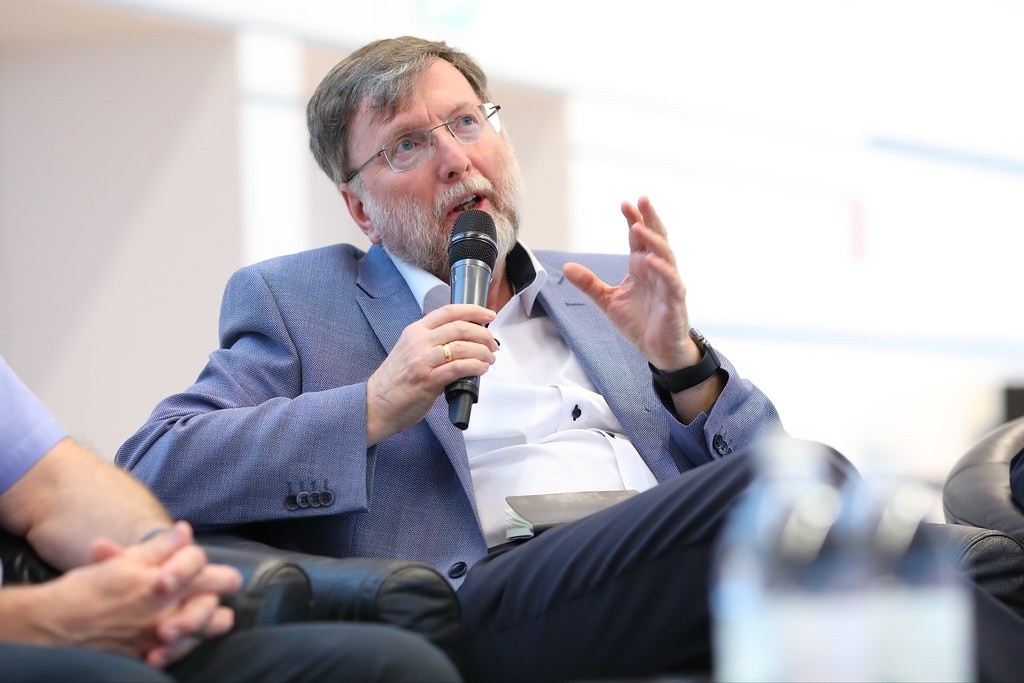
[940,417,1024,543]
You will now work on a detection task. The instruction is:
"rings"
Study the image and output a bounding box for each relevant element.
[442,343,453,362]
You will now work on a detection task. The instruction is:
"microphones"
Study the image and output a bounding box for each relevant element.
[444,210,498,430]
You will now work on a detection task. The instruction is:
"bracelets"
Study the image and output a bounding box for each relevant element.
[648,328,721,393]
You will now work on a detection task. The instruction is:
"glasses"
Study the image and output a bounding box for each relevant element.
[344,103,502,183]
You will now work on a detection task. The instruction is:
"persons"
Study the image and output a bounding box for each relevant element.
[116,34,1023,681]
[0,356,463,681]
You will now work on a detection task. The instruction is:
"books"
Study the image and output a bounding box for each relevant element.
[505,490,640,540]
